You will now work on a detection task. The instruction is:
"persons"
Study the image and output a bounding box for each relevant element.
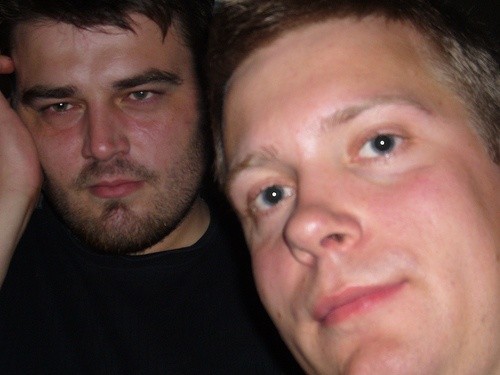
[0,0,312,374]
[211,0,500,374]
